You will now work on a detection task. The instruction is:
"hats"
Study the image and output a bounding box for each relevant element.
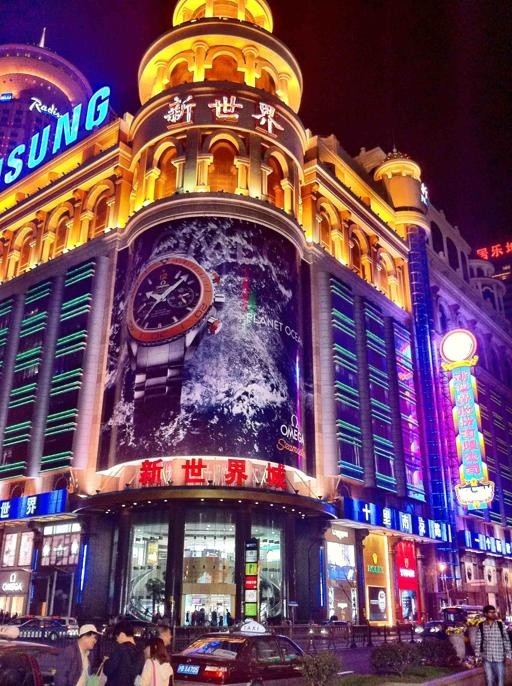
[78,624,104,637]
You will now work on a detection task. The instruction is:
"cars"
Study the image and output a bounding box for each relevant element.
[446,612,487,635]
[416,620,460,640]
[308,621,351,640]
[0,614,81,646]
[170,622,328,686]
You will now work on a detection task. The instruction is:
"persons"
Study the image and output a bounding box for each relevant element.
[154,625,175,686]
[0,609,19,626]
[473,604,512,686]
[52,622,104,686]
[137,638,173,686]
[184,608,235,633]
[103,621,144,686]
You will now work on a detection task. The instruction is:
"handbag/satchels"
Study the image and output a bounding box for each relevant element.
[87,660,107,686]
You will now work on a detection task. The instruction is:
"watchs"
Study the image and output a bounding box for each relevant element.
[123,249,226,442]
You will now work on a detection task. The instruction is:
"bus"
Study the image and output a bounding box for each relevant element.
[439,603,483,627]
